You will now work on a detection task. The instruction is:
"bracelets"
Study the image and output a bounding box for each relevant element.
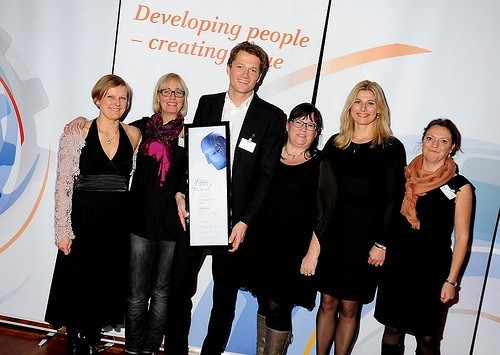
[374,243,386,250]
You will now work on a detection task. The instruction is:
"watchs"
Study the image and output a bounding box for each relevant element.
[446,279,460,292]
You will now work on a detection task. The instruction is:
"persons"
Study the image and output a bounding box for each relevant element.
[63,73,189,355]
[316,80,406,355]
[44,74,143,355]
[373,118,472,355]
[162,40,287,355]
[240,102,321,355]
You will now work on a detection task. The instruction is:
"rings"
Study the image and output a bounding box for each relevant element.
[301,272,304,275]
[308,272,311,274]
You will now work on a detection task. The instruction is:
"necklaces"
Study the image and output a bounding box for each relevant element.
[98,124,119,144]
[353,133,373,153]
[285,144,308,159]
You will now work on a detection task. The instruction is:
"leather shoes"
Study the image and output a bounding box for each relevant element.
[67,337,78,355]
[85,343,96,355]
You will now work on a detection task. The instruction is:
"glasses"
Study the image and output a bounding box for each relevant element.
[290,119,318,131]
[158,89,185,98]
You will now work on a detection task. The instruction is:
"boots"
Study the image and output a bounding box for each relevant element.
[263,327,291,355]
[255,313,266,355]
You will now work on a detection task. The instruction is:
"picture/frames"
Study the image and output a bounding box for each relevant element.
[184,121,233,251]
